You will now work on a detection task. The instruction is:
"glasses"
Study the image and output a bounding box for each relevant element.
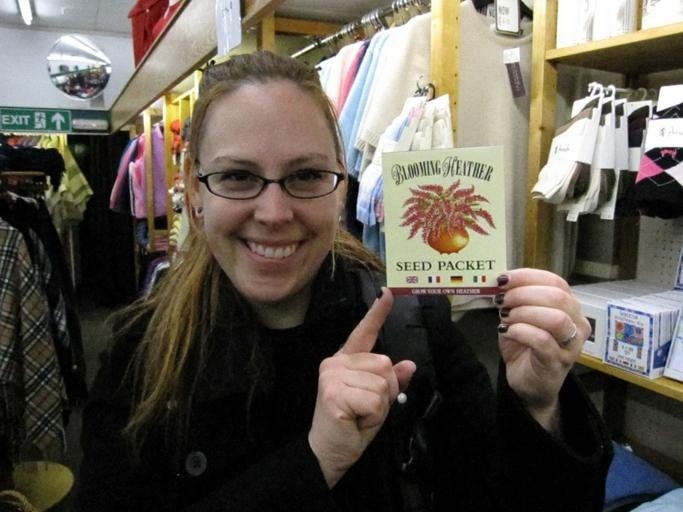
[195,167,346,200]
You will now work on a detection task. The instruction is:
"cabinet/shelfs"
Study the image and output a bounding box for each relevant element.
[526,0,683,403]
[126,67,199,252]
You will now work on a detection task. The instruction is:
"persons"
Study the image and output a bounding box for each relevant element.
[82,50,614,512]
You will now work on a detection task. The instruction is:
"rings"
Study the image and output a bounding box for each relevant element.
[561,323,580,345]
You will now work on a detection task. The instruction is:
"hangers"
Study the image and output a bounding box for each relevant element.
[313,2,426,54]
